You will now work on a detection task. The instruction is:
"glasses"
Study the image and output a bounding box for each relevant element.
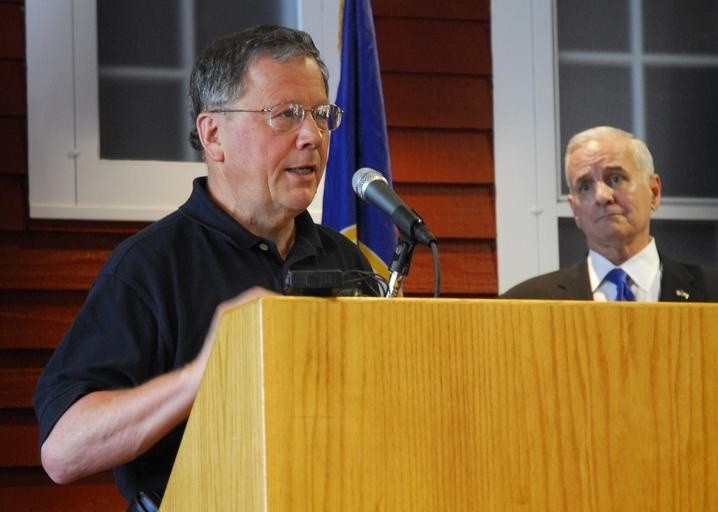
[209,102,343,134]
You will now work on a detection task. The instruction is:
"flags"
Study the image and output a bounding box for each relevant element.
[318,2,407,297]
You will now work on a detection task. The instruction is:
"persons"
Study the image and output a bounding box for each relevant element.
[32,21,384,511]
[492,125,718,305]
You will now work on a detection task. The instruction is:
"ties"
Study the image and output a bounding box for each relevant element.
[605,269,637,305]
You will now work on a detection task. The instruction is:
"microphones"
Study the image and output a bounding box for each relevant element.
[351,166,437,247]
